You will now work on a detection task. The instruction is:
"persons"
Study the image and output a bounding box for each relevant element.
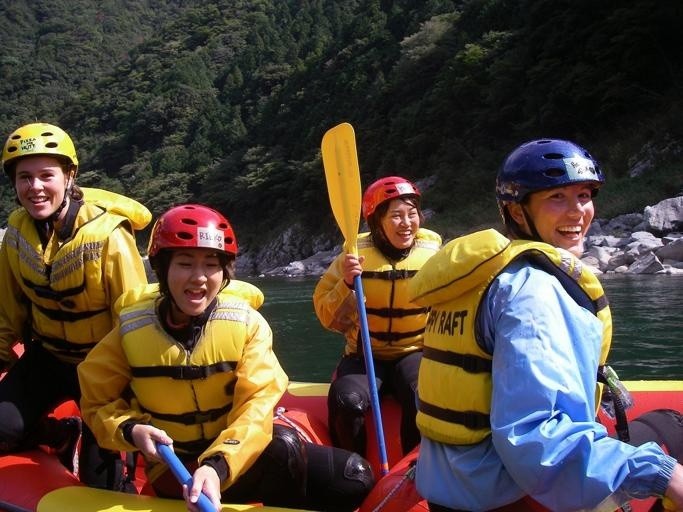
[407,139,683,512]
[312,176,442,464]
[0,122,156,490]
[78,204,373,512]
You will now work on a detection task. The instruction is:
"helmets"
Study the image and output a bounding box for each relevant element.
[1,122,79,179]
[362,176,421,226]
[147,204,240,272]
[495,138,607,226]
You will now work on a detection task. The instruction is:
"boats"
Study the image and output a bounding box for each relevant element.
[0,336,683,512]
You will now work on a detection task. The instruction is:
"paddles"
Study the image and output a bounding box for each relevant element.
[321,122,390,476]
